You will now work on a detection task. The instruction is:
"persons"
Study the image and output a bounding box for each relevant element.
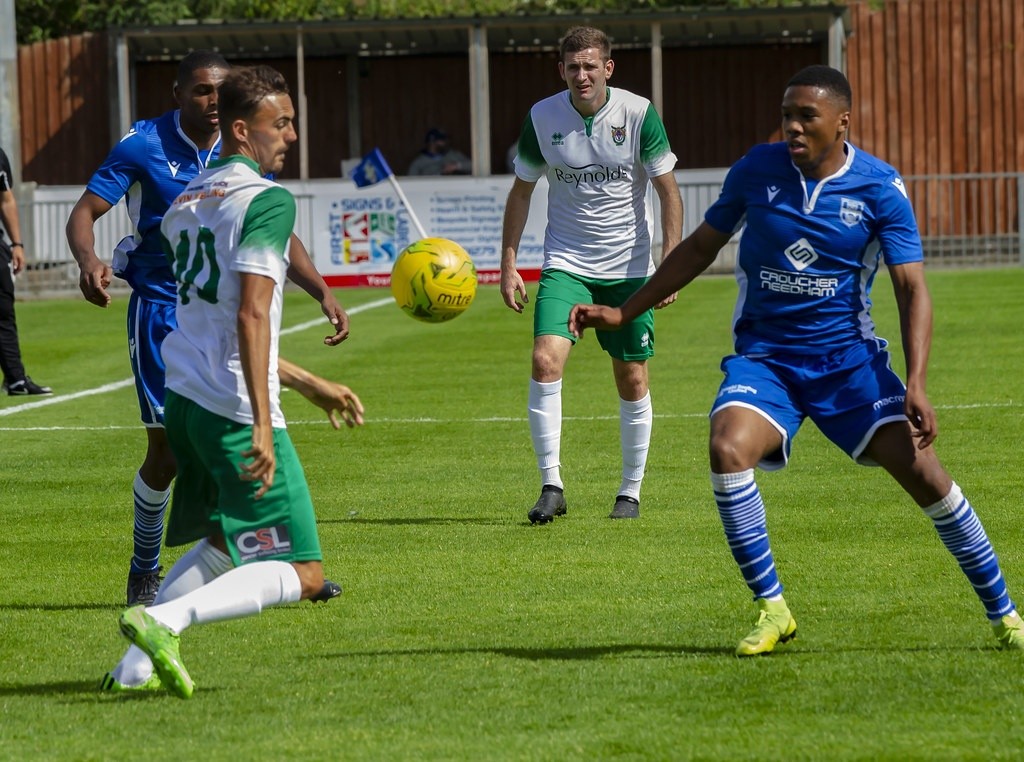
[568,63,1023,658]
[115,66,366,698]
[407,128,471,176]
[499,25,682,523]
[65,46,348,697]
[1,147,53,396]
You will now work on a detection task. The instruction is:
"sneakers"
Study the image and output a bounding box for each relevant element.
[608,496,641,519]
[124,556,161,607]
[734,597,798,657]
[309,582,340,602]
[97,671,164,692]
[992,618,1024,648]
[119,608,196,699]
[3,377,51,396]
[529,485,566,522]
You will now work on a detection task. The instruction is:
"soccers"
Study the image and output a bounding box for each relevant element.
[390,237,478,323]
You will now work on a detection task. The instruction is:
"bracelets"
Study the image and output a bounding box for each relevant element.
[11,243,24,248]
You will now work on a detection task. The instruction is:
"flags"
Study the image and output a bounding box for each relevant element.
[347,147,394,189]
